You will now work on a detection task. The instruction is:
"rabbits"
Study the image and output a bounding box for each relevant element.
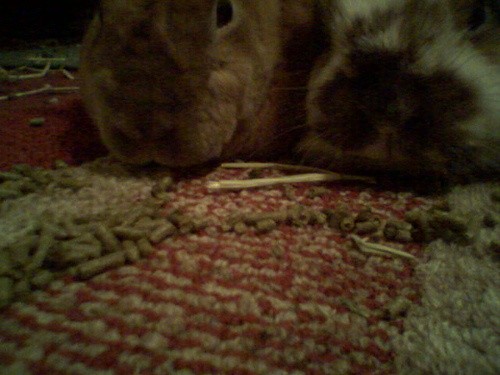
[78,0,500,184]
